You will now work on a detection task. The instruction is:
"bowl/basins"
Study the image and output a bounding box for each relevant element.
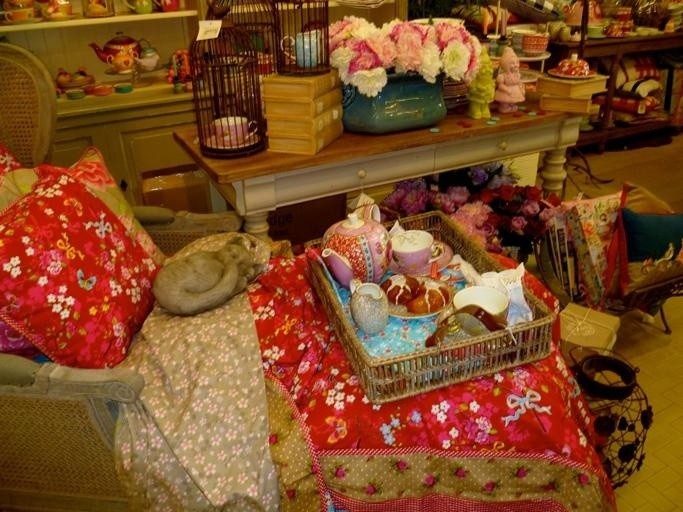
[54,75,96,94]
[510,29,550,57]
[65,82,133,100]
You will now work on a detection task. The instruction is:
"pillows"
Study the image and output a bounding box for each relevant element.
[0,139,169,370]
[620,207,683,261]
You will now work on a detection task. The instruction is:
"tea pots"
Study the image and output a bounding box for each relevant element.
[320,204,393,290]
[87,31,160,76]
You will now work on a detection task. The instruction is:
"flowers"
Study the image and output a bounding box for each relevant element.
[377,160,562,250]
[325,14,478,86]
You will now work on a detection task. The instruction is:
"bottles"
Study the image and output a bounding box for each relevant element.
[172,72,204,94]
[549,22,570,42]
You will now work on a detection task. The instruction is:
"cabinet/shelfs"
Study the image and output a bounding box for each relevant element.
[1,1,406,209]
[532,2,683,159]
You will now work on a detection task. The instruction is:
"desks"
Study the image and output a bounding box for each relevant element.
[172,98,600,239]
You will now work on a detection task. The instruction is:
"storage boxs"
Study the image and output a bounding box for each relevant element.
[559,303,620,365]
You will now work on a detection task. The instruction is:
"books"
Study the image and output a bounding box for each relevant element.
[536,75,609,113]
[261,69,344,156]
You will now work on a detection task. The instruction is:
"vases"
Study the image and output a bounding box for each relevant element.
[339,70,450,134]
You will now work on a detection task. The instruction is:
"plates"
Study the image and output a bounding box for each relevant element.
[203,133,260,151]
[378,276,454,319]
[586,36,607,39]
[383,239,453,276]
[547,70,596,80]
[519,69,547,85]
[622,27,663,37]
[484,43,552,62]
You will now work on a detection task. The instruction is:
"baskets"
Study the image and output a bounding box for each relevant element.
[303,210,557,406]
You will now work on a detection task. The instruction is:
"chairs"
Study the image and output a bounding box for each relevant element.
[2,45,59,167]
[0,148,620,512]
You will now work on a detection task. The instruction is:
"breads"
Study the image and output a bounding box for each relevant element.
[380,273,421,306]
[407,281,451,314]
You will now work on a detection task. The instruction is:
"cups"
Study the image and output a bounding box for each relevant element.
[2,0,181,24]
[587,26,603,36]
[279,30,322,70]
[392,229,444,270]
[600,3,634,37]
[348,278,389,335]
[211,118,258,146]
[454,286,510,336]
